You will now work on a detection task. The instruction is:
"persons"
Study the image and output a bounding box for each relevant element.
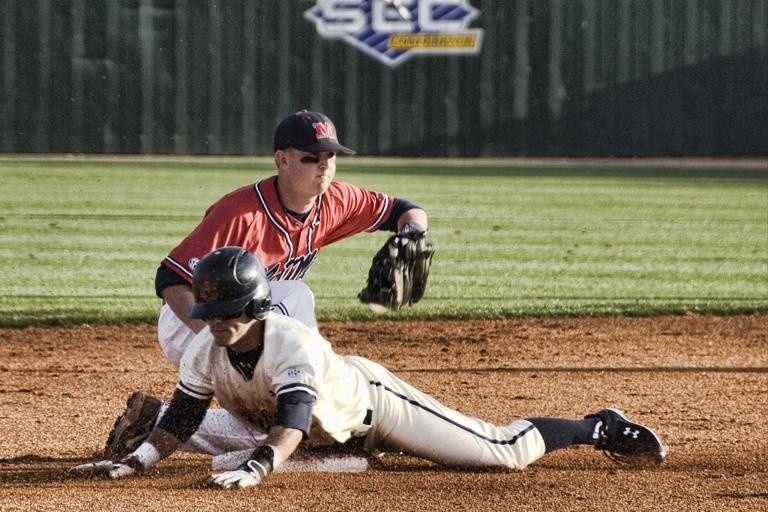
[155,109,434,369]
[69,246,666,490]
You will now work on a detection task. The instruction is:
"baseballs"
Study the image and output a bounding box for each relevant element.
[369,303,388,316]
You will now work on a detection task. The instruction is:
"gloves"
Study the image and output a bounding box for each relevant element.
[70,441,162,478]
[212,445,277,488]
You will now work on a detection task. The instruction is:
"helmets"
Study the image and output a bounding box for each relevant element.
[274,109,356,155]
[188,245,271,320]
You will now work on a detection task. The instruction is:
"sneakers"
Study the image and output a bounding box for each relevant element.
[103,391,161,462]
[583,408,666,460]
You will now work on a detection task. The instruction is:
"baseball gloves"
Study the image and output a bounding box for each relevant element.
[356,224,435,313]
